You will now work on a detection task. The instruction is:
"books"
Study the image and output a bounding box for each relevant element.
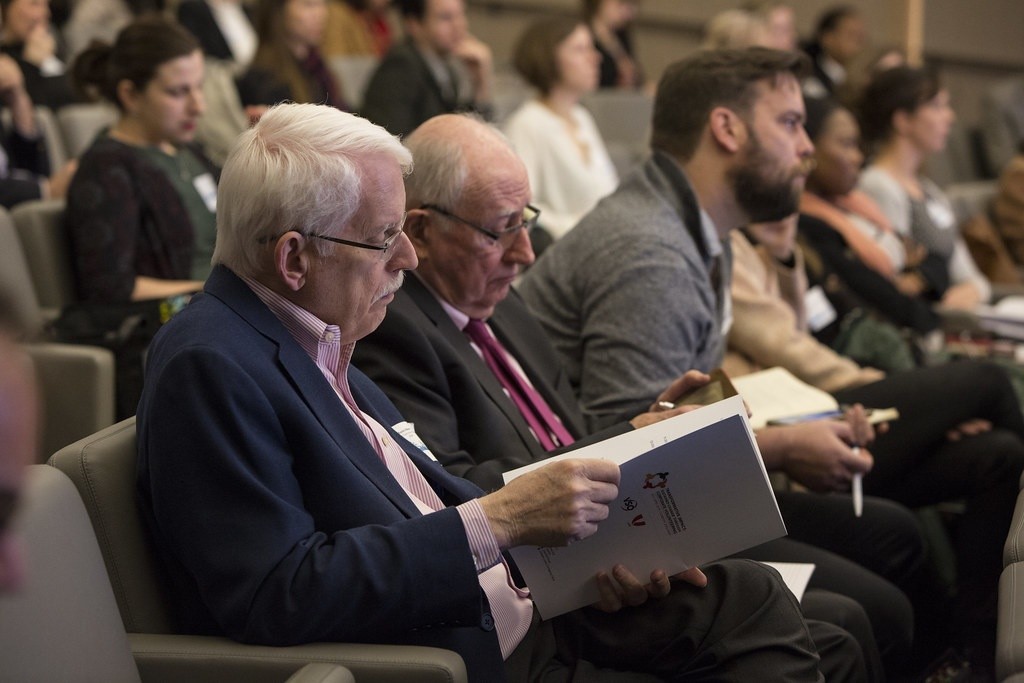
[502,394,787,621]
[731,365,840,428]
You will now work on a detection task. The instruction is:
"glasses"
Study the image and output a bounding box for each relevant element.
[258,210,407,253]
[419,203,540,243]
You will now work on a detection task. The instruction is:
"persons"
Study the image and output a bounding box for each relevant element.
[0,1,1024,683]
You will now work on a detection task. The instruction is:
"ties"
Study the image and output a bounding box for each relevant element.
[463,315,575,451]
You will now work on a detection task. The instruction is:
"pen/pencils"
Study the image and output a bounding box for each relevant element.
[850,444,864,517]
[658,401,758,438]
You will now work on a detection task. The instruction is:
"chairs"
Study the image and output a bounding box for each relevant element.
[0,56,1024,683]
